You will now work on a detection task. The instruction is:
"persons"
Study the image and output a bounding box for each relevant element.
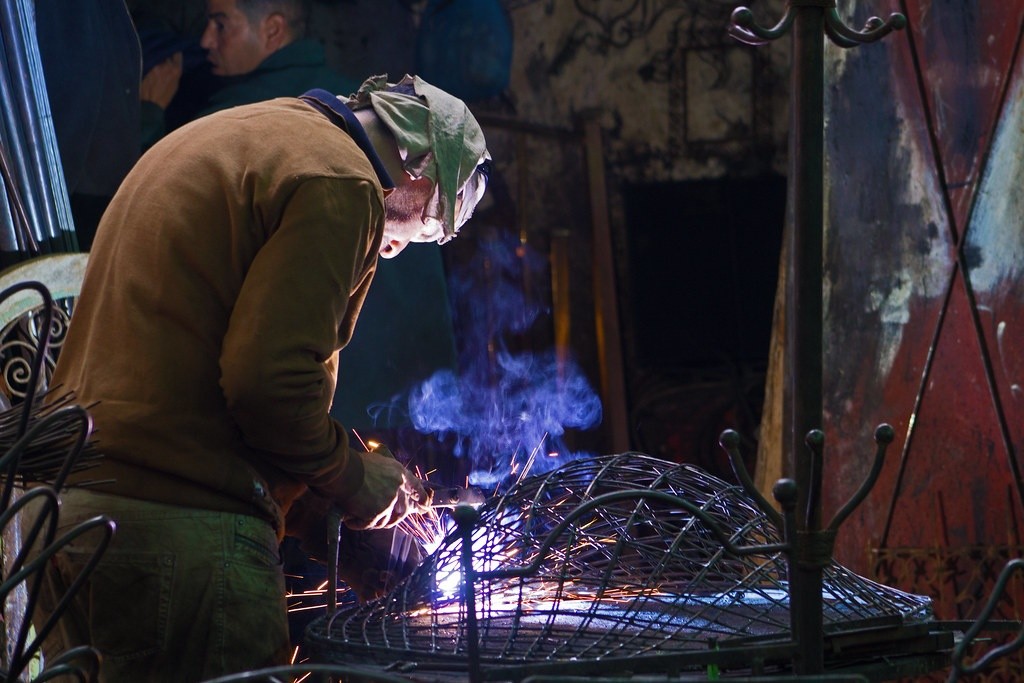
[15,74,495,683]
[135,0,468,498]
